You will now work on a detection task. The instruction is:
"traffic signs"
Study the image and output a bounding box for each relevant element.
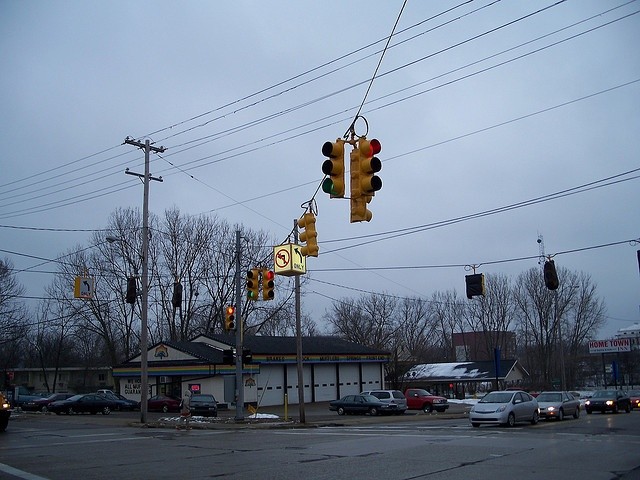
[75,277,93,299]
[291,244,305,274]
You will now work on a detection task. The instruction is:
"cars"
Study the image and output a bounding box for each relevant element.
[568,390,590,409]
[584,390,630,414]
[47,393,118,416]
[137,393,184,412]
[469,390,539,428]
[328,394,389,416]
[31,393,77,414]
[189,393,219,417]
[0,392,12,433]
[535,391,580,421]
[626,389,640,411]
[98,392,139,411]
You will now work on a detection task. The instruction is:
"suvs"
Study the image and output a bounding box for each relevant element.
[405,388,449,413]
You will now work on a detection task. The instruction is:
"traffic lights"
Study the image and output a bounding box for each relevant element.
[263,268,275,301]
[225,306,234,330]
[466,273,485,298]
[171,282,182,307]
[349,136,382,224]
[543,260,559,290]
[320,140,345,199]
[297,212,319,257]
[126,278,136,304]
[246,269,258,299]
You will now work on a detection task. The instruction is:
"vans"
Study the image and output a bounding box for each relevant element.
[359,389,409,416]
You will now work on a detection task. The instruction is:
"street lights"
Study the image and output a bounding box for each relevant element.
[106,235,148,424]
[556,285,580,390]
[235,231,252,420]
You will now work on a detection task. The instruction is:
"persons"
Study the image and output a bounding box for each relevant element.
[176,390,194,430]
